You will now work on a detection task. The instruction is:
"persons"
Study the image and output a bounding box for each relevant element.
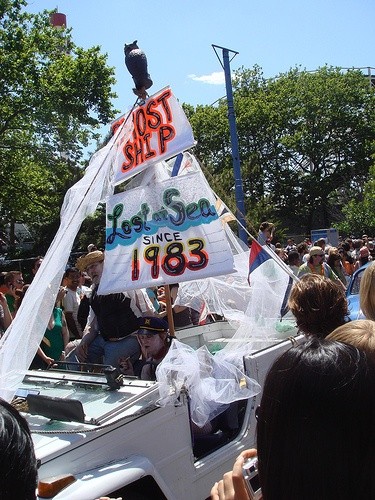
[144,283,210,331]
[290,274,347,339]
[0,397,37,500]
[64,250,156,373]
[0,256,96,372]
[260,222,288,261]
[274,235,375,291]
[359,260,375,321]
[118,317,177,381]
[325,319,375,352]
[210,337,375,500]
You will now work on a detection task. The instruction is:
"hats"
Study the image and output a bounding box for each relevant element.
[309,246,325,256]
[134,316,167,335]
[359,246,370,258]
[76,251,104,272]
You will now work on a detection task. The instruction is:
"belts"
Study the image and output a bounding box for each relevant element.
[109,329,139,341]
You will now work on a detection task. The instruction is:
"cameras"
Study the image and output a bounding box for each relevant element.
[242,456,263,500]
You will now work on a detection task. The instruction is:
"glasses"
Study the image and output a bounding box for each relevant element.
[315,255,323,257]
[86,263,101,273]
[37,264,40,267]
[9,279,24,284]
[254,405,261,421]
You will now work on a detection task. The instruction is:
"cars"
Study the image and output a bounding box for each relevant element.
[0,284,312,500]
[344,260,375,323]
